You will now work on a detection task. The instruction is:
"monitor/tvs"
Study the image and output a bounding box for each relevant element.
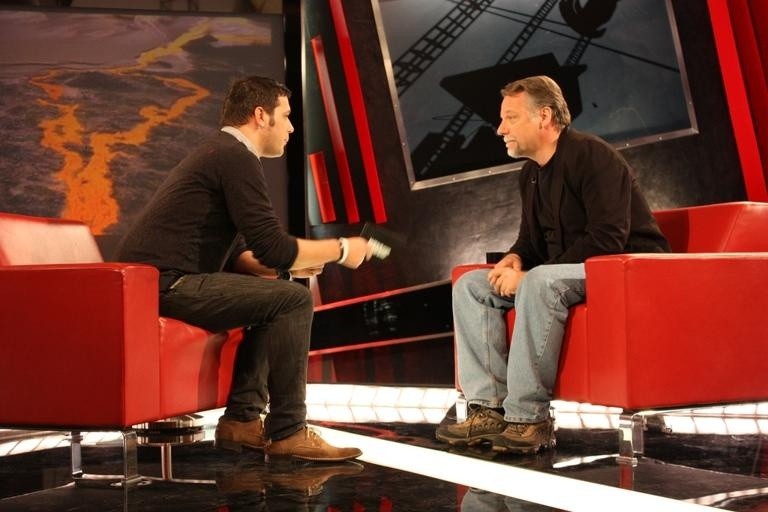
[372,0,700,193]
[0,0,309,288]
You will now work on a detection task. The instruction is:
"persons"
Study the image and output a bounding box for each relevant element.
[434,74,669,456]
[109,76,373,461]
[213,450,368,511]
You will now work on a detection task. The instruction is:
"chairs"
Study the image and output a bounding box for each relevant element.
[451,198,767,458]
[0,209,255,488]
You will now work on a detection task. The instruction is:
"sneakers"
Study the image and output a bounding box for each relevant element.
[436,408,508,447]
[492,415,557,455]
[214,415,268,453]
[265,422,362,464]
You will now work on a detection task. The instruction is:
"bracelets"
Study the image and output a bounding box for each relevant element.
[335,237,350,265]
[276,270,293,281]
